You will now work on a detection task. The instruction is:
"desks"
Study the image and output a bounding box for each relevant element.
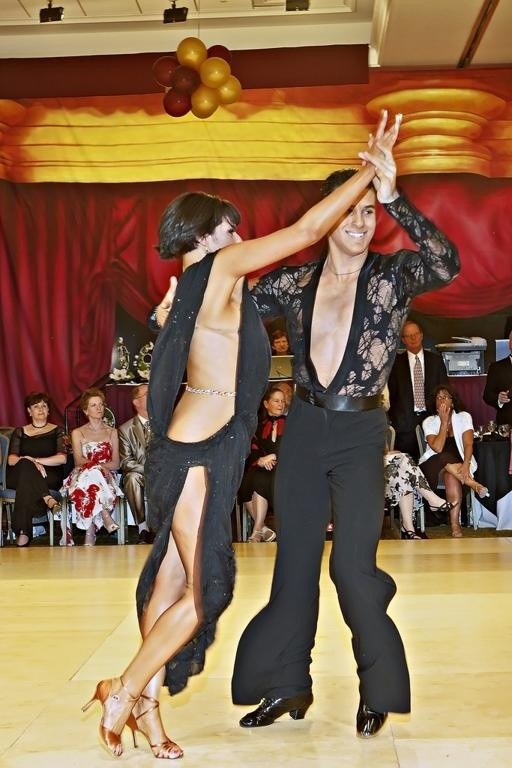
[468,434,512,531]
[446,374,498,429]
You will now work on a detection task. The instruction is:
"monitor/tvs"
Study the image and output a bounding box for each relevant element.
[270,354,295,379]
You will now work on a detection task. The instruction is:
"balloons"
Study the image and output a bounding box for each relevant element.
[152,37,242,119]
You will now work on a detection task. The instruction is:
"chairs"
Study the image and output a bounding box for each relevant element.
[416,424,462,533]
[232,494,243,543]
[0,433,13,544]
[243,503,253,543]
[384,424,396,530]
[61,405,121,547]
[121,494,146,545]
[0,489,61,546]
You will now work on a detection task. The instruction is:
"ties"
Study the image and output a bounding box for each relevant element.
[413,354,426,410]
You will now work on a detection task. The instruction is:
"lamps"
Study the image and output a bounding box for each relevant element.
[286,0,310,12]
[163,0,189,23]
[39,0,66,22]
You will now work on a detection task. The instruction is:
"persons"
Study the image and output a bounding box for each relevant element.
[147,143,461,738]
[117,384,152,544]
[6,391,76,547]
[59,387,125,546]
[237,389,288,543]
[272,382,293,416]
[382,320,512,539]
[270,330,294,355]
[82,108,403,759]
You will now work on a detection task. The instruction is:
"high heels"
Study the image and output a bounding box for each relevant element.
[429,499,459,521]
[80,673,141,759]
[474,482,491,500]
[45,496,62,519]
[126,693,185,760]
[239,693,314,728]
[399,524,420,539]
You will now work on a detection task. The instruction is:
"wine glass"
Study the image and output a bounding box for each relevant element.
[478,421,510,442]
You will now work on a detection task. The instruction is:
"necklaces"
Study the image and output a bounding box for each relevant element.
[326,258,362,275]
[31,421,48,428]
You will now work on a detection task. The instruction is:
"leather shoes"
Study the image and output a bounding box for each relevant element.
[356,700,389,739]
[135,530,152,545]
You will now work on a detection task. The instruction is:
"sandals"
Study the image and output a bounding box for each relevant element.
[82,532,98,547]
[262,525,276,542]
[103,519,120,536]
[245,529,262,544]
[16,532,31,548]
[450,521,463,538]
